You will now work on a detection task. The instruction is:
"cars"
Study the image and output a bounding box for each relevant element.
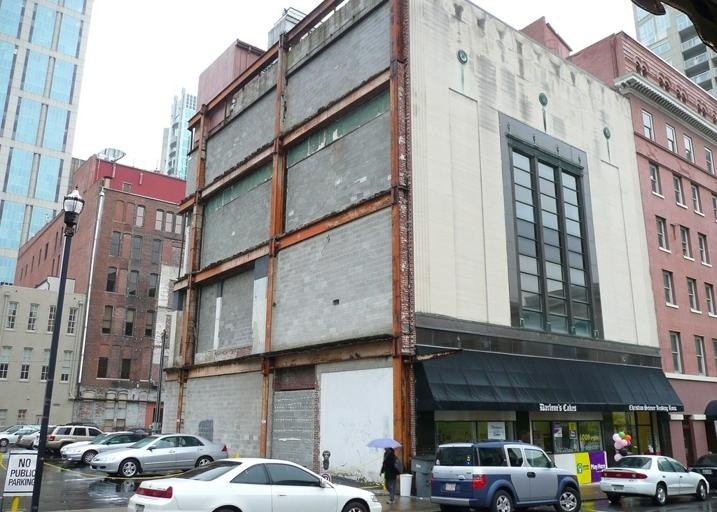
[599,453,717,506]
[430,439,581,512]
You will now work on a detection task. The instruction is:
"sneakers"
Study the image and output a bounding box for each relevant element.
[386,495,399,505]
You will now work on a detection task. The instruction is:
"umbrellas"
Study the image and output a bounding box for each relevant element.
[366,437,402,449]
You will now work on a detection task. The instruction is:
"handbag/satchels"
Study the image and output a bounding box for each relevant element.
[394,457,403,475]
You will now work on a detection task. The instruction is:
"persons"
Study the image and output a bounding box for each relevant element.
[379,447,400,503]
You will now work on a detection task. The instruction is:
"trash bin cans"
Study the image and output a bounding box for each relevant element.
[410,454,436,497]
[399,474,413,497]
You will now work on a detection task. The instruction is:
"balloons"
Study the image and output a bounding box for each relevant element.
[612,431,632,461]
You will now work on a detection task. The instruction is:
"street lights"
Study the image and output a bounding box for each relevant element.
[30,183,84,511]
